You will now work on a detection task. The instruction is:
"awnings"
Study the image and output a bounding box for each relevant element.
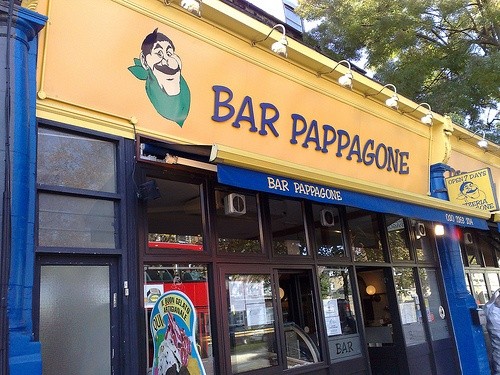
[169,143,491,230]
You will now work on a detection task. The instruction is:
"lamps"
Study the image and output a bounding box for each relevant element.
[317,59,353,85]
[365,84,399,107]
[458,129,488,147]
[164,0,201,17]
[251,24,288,57]
[401,103,433,125]
[366,284,376,295]
